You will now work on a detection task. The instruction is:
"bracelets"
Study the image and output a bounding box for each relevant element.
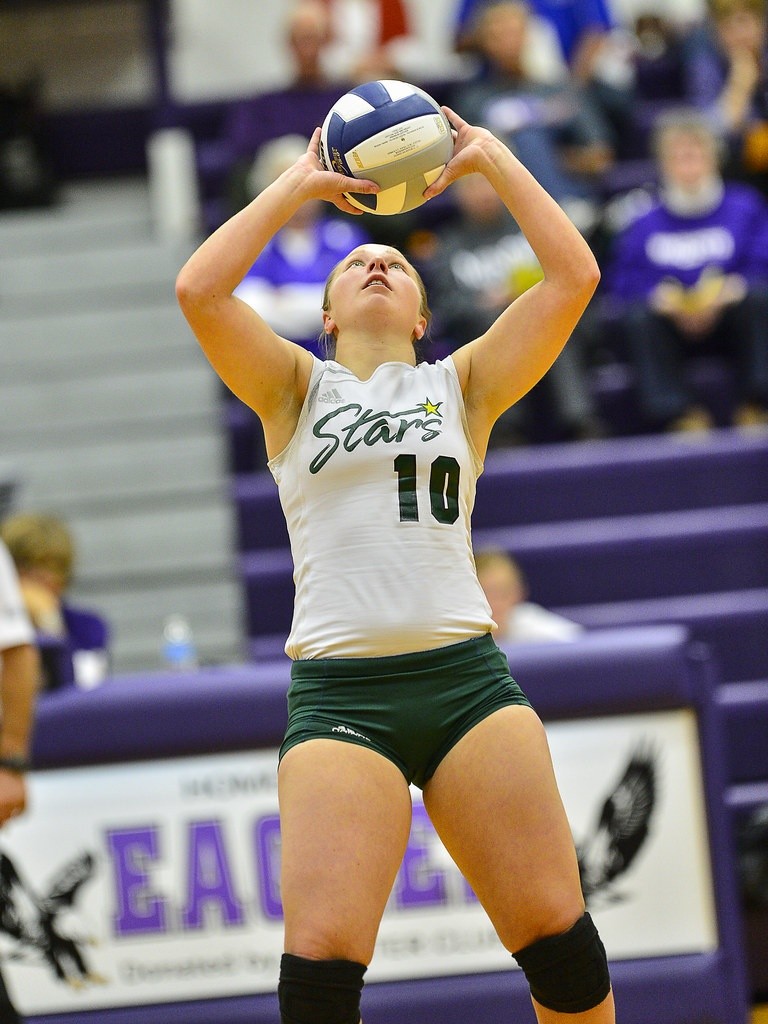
[1,753,30,773]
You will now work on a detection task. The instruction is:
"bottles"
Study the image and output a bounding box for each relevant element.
[163,613,197,676]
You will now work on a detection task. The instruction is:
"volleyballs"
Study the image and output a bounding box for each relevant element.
[318,77,454,215]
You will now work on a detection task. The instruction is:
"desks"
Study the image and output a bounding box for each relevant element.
[0,621,751,1024]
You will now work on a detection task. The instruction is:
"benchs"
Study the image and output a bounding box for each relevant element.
[197,90,768,848]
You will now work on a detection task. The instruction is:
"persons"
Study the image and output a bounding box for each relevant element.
[235,0,768,455]
[0,505,113,827]
[171,105,619,1024]
[470,541,591,645]
